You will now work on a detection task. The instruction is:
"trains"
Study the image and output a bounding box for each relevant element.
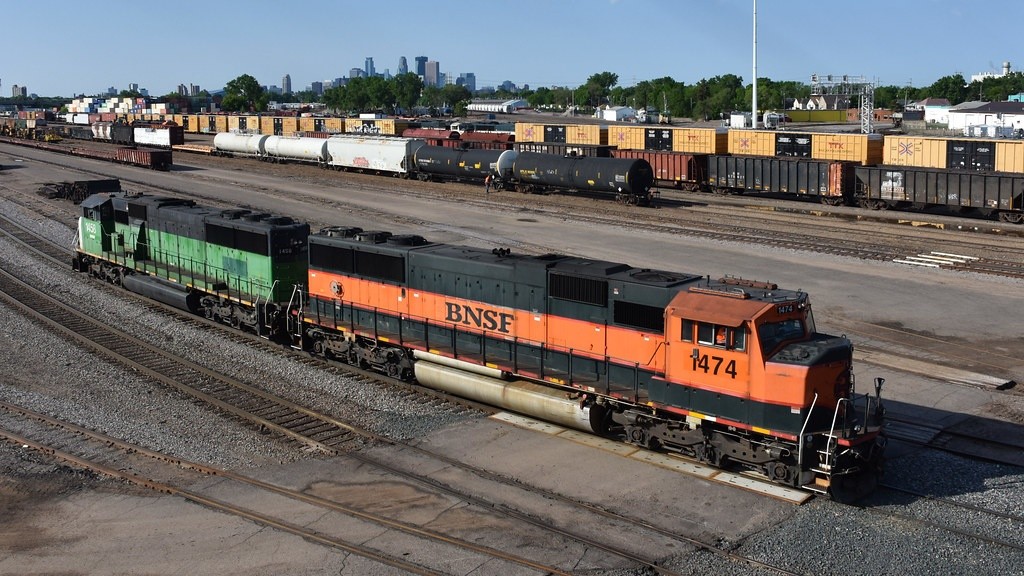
[213,132,656,208]
[73,191,892,502]
[0,111,1024,234]
